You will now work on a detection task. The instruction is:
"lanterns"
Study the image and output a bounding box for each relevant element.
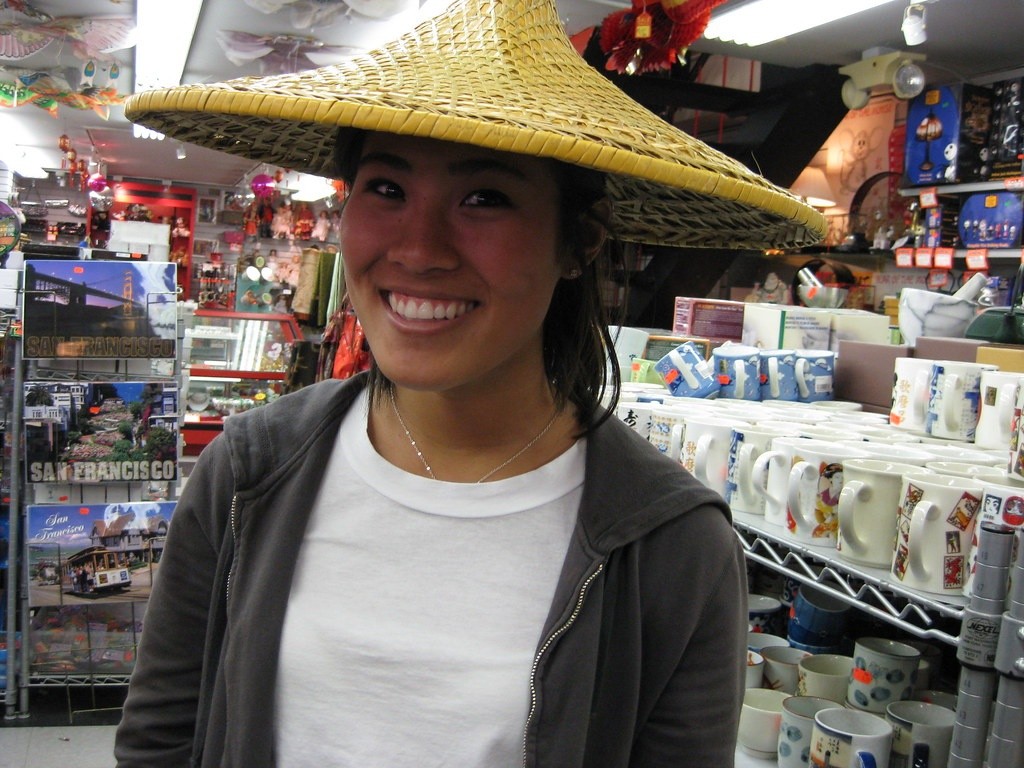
[58,134,89,190]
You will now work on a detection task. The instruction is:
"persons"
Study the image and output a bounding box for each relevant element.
[244,196,340,288]
[114,122,746,768]
[171,217,191,263]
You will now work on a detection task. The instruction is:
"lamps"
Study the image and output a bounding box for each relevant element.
[176,143,186,159]
[89,145,97,166]
[901,0,938,46]
[838,46,940,111]
[324,196,333,208]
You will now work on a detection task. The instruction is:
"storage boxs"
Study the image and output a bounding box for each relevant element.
[903,83,996,184]
[926,204,960,247]
[674,296,890,359]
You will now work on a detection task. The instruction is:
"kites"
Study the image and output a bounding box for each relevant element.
[0,0,137,121]
[218,0,408,77]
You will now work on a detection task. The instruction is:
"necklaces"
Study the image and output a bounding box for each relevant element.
[390,380,562,483]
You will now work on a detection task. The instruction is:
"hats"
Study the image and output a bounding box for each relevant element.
[124,0,827,252]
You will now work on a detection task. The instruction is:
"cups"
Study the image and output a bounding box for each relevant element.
[737,584,958,768]
[597,341,1024,596]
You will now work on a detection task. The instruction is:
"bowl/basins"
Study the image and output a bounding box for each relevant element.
[799,285,849,308]
[898,287,978,344]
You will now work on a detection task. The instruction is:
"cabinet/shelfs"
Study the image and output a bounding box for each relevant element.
[0,288,180,720]
[730,507,1024,768]
[87,180,198,302]
[181,309,304,456]
[897,181,1024,258]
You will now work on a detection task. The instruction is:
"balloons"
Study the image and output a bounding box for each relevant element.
[88,173,106,192]
[251,174,275,199]
